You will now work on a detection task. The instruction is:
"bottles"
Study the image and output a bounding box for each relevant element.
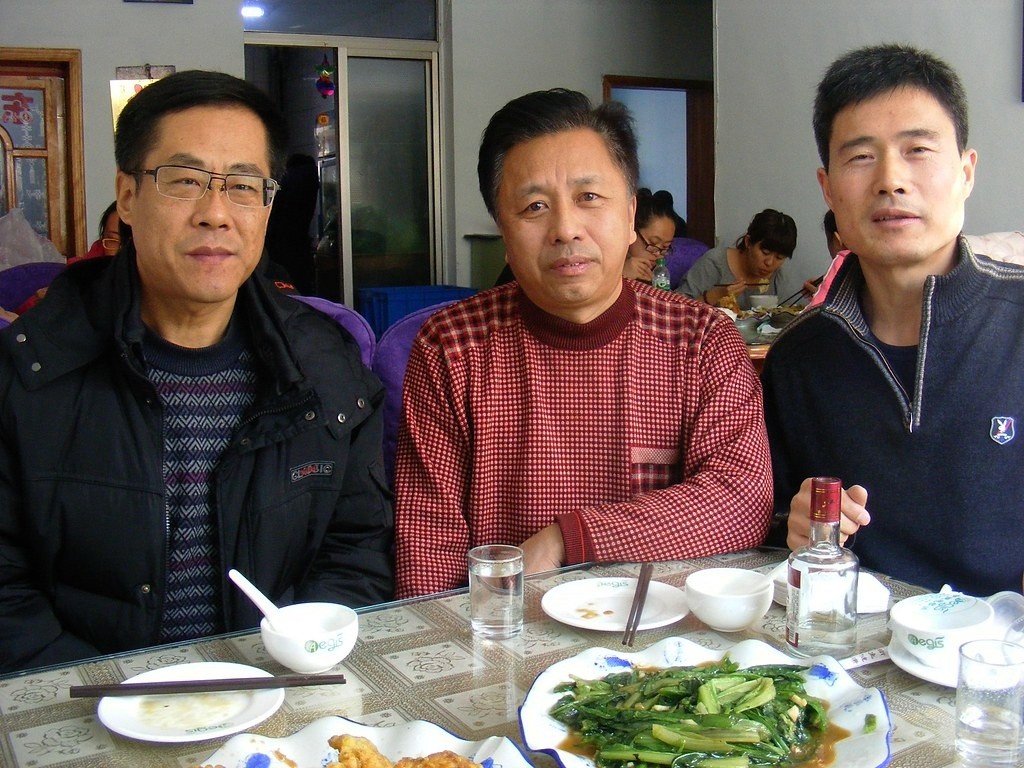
[651,259,670,291]
[786,476,859,661]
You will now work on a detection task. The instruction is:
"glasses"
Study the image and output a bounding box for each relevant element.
[636,227,671,257]
[128,165,281,208]
[102,238,124,251]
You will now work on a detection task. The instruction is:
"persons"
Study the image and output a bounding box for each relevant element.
[1,71,393,674]
[759,46,1024,598]
[622,199,676,286]
[12,200,120,317]
[636,188,686,238]
[674,210,797,311]
[801,210,849,301]
[394,87,773,600]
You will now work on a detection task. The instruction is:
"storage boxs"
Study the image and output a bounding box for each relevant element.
[357,284,478,344]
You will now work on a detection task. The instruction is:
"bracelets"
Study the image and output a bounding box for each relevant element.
[703,290,717,307]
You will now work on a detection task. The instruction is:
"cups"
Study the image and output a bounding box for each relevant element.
[956,639,1024,767]
[468,544,523,639]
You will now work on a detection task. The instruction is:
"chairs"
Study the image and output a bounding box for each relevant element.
[0,258,465,509]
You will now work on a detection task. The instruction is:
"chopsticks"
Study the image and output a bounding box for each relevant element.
[714,283,770,286]
[70,674,347,698]
[777,275,824,308]
[622,563,653,646]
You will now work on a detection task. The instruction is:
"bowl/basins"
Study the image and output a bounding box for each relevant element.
[686,567,775,632]
[261,602,359,674]
[891,592,995,667]
[749,295,778,308]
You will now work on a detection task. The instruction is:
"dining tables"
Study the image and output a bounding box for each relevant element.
[0,542,1024,768]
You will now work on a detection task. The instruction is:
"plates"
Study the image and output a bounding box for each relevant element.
[889,639,1023,690]
[771,312,797,323]
[736,309,772,322]
[97,662,286,743]
[541,578,691,632]
[517,638,891,768]
[196,716,534,768]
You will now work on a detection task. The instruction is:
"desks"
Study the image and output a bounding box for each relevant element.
[733,307,773,375]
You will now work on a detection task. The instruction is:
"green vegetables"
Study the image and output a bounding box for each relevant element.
[547,652,828,768]
